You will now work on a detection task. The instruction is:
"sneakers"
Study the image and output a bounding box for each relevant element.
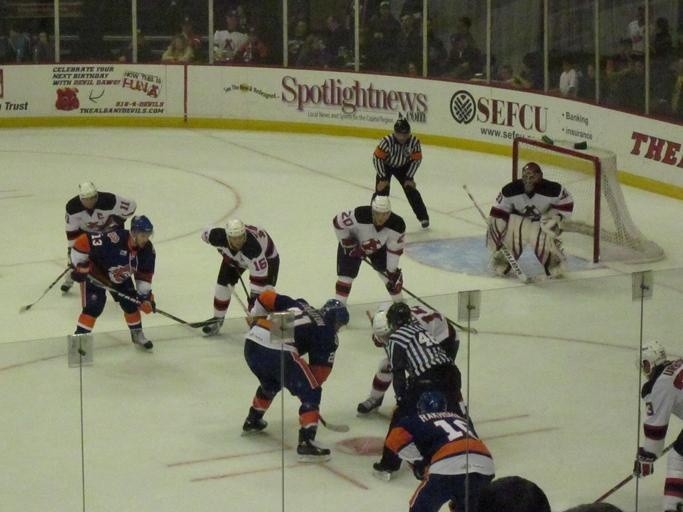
[203,317,223,336]
[373,459,391,473]
[61,277,72,290]
[244,413,268,430]
[130,328,154,347]
[75,329,89,356]
[296,440,332,455]
[357,394,383,412]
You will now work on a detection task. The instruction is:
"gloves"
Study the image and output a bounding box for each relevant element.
[71,262,90,282]
[387,268,403,294]
[346,245,366,259]
[633,449,656,478]
[136,290,156,314]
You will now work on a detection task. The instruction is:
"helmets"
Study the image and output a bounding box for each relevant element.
[636,341,665,379]
[416,391,447,413]
[131,216,153,248]
[522,163,543,192]
[372,196,391,226]
[225,220,247,250]
[79,182,99,210]
[322,300,348,332]
[372,303,410,338]
[394,119,410,144]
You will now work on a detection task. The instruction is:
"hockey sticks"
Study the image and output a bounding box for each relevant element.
[462,185,548,283]
[87,275,218,328]
[226,283,349,431]
[361,255,477,334]
[19,267,71,312]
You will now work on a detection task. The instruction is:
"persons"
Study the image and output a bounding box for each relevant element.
[1,0,681,126]
[636,340,683,512]
[366,303,626,512]
[487,163,574,270]
[69,213,157,349]
[200,219,280,333]
[331,194,407,307]
[241,291,349,457]
[60,180,137,292]
[371,120,429,229]
